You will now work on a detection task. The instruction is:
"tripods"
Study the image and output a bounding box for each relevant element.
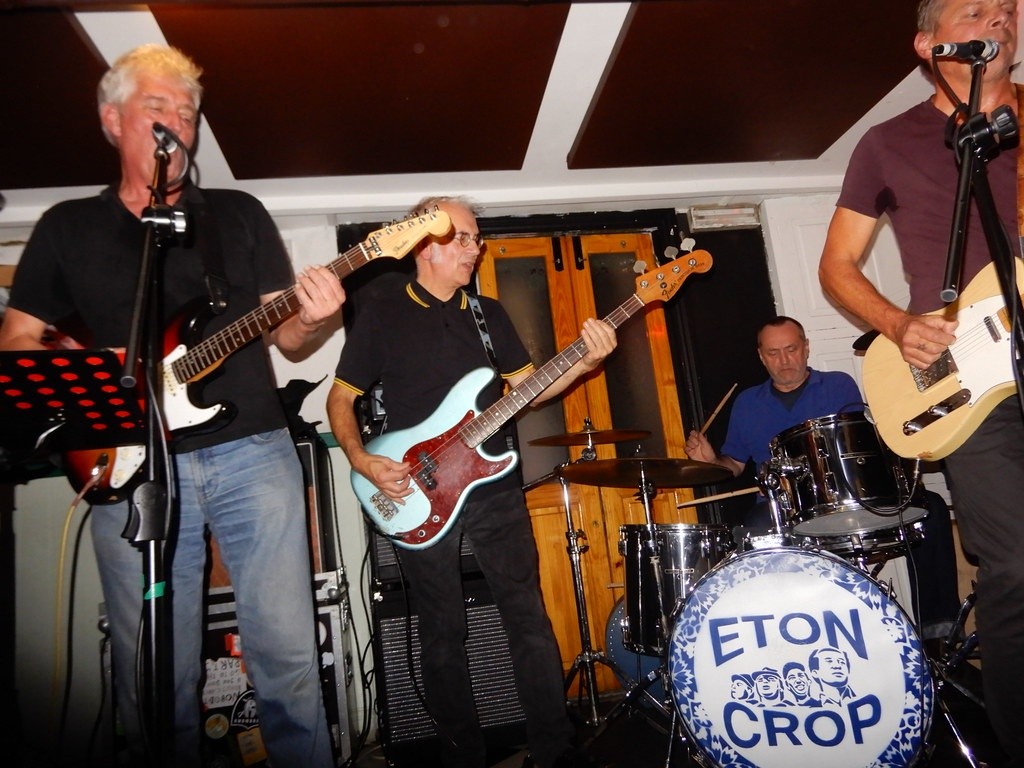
[522,420,684,768]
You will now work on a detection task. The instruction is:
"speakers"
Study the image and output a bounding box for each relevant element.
[361,425,528,762]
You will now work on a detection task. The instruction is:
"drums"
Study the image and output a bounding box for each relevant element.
[664,533,942,768]
[616,521,739,658]
[767,409,934,539]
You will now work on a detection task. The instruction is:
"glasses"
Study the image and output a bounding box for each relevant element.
[445,233,483,248]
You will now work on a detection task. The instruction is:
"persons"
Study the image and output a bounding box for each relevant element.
[817,0,1024,767]
[0,48,347,768]
[684,316,961,652]
[327,197,618,768]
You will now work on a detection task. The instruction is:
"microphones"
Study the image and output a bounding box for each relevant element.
[152,121,177,154]
[933,39,999,62]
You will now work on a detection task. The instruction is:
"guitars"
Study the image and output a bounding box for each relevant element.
[348,237,715,552]
[56,204,455,508]
[860,253,1024,463]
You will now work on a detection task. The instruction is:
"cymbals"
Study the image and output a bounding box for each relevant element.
[553,456,735,491]
[526,428,653,448]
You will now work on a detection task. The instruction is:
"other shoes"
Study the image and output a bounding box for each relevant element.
[523,747,594,768]
